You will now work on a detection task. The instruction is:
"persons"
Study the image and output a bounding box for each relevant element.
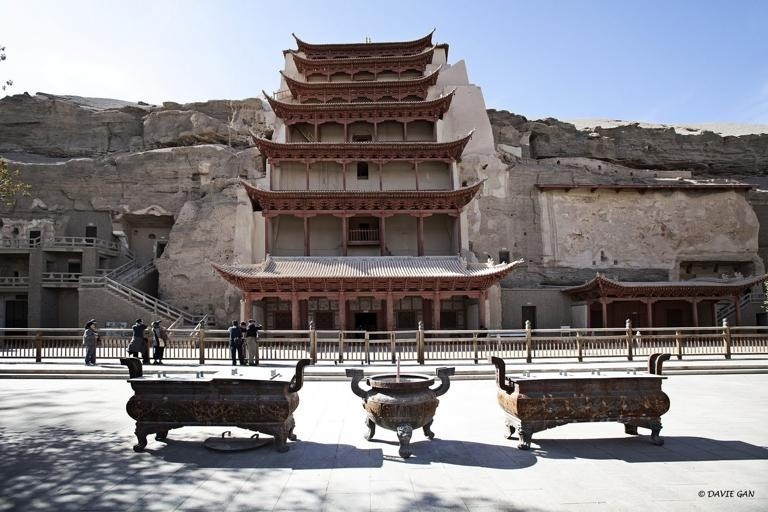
[228,319,263,366]
[477,326,487,345]
[132,319,151,364]
[151,320,167,364]
[83,322,97,366]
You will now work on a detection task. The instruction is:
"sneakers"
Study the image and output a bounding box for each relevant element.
[86,361,95,366]
[233,361,258,366]
[154,362,162,365]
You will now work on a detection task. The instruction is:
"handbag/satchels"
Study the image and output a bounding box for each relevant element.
[234,337,242,346]
[158,337,164,347]
